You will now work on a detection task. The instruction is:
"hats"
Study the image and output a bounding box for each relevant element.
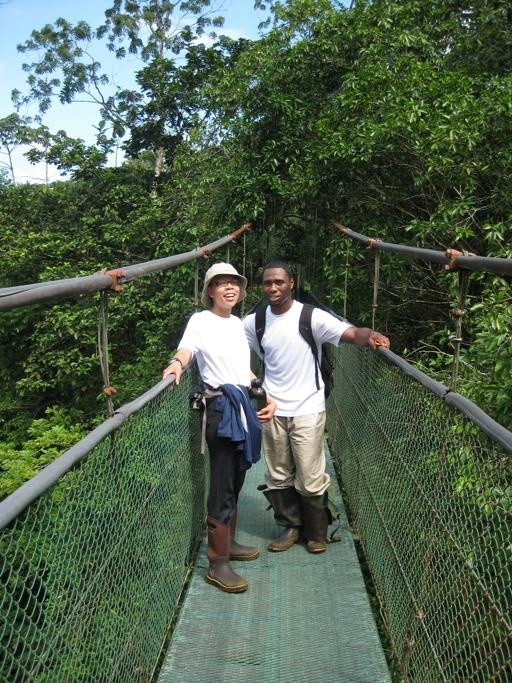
[201,262,248,308]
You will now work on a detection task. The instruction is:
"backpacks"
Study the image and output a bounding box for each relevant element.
[255,303,335,398]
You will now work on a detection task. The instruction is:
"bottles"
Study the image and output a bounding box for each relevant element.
[249,378,267,422]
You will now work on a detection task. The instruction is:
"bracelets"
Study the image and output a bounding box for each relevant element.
[167,357,183,367]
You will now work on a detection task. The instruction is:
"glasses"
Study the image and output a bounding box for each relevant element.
[213,279,240,287]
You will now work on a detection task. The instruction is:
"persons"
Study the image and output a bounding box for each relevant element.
[162,262,277,594]
[242,261,390,553]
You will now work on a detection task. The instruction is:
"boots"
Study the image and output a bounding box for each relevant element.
[263,487,302,552]
[296,489,328,553]
[230,510,258,561]
[205,517,248,593]
